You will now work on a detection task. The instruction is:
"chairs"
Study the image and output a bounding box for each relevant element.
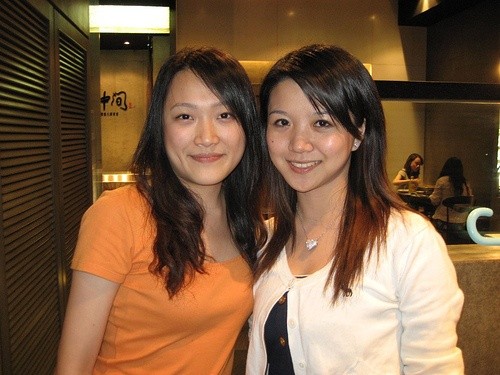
[441,196,475,244]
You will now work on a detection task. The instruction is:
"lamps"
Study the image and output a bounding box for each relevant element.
[88,4,170,34]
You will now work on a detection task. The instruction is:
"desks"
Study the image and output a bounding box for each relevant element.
[397,192,433,218]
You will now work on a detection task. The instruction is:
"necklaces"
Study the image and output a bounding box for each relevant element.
[294,207,342,251]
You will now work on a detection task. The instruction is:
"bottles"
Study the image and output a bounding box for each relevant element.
[409,176,416,194]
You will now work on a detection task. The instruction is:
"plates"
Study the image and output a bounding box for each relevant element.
[416,191,424,194]
[398,190,409,192]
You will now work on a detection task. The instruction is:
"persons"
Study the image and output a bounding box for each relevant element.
[430,156,473,234]
[54,48,262,375]
[393,154,429,215]
[245,44,467,375]
